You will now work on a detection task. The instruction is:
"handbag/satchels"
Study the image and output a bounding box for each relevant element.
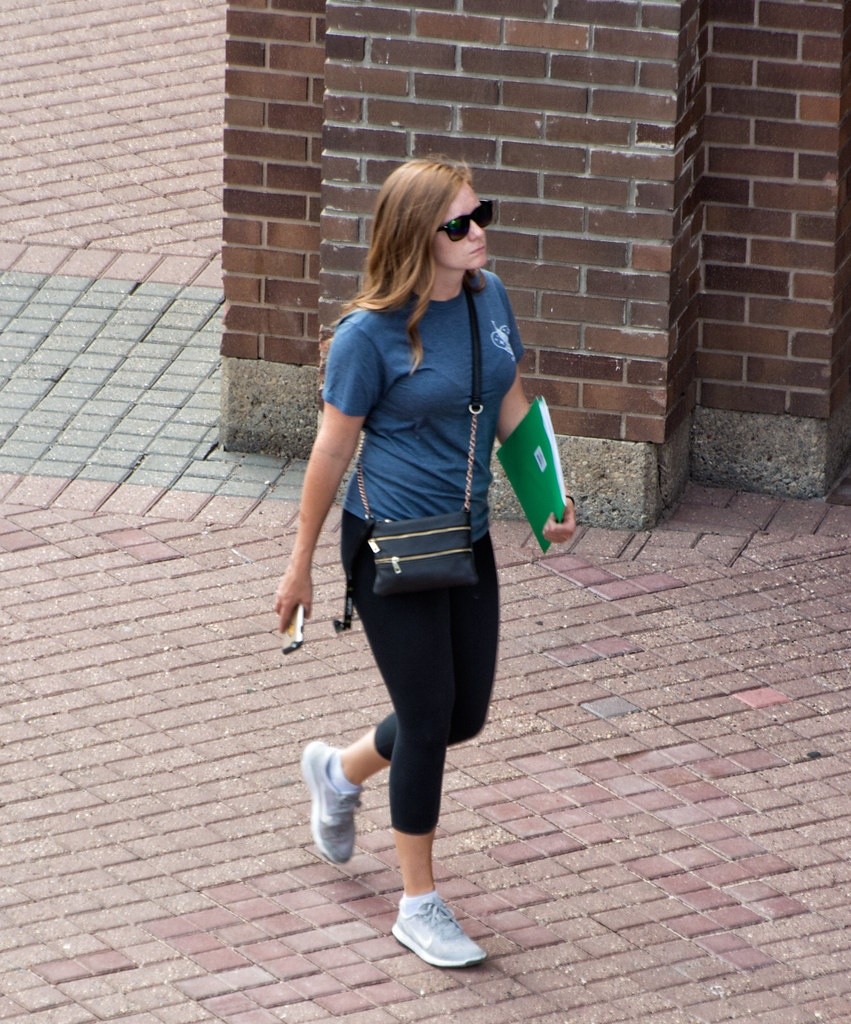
[367,510,482,597]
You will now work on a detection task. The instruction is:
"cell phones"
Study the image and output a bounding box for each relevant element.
[281,602,306,654]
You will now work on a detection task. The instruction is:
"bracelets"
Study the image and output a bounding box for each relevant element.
[566,495,575,506]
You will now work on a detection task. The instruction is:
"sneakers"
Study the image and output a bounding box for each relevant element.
[302,740,363,864]
[392,898,487,968]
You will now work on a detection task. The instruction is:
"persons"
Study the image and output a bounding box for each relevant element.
[274,155,576,968]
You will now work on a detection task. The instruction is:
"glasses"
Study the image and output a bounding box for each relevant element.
[438,199,495,242]
